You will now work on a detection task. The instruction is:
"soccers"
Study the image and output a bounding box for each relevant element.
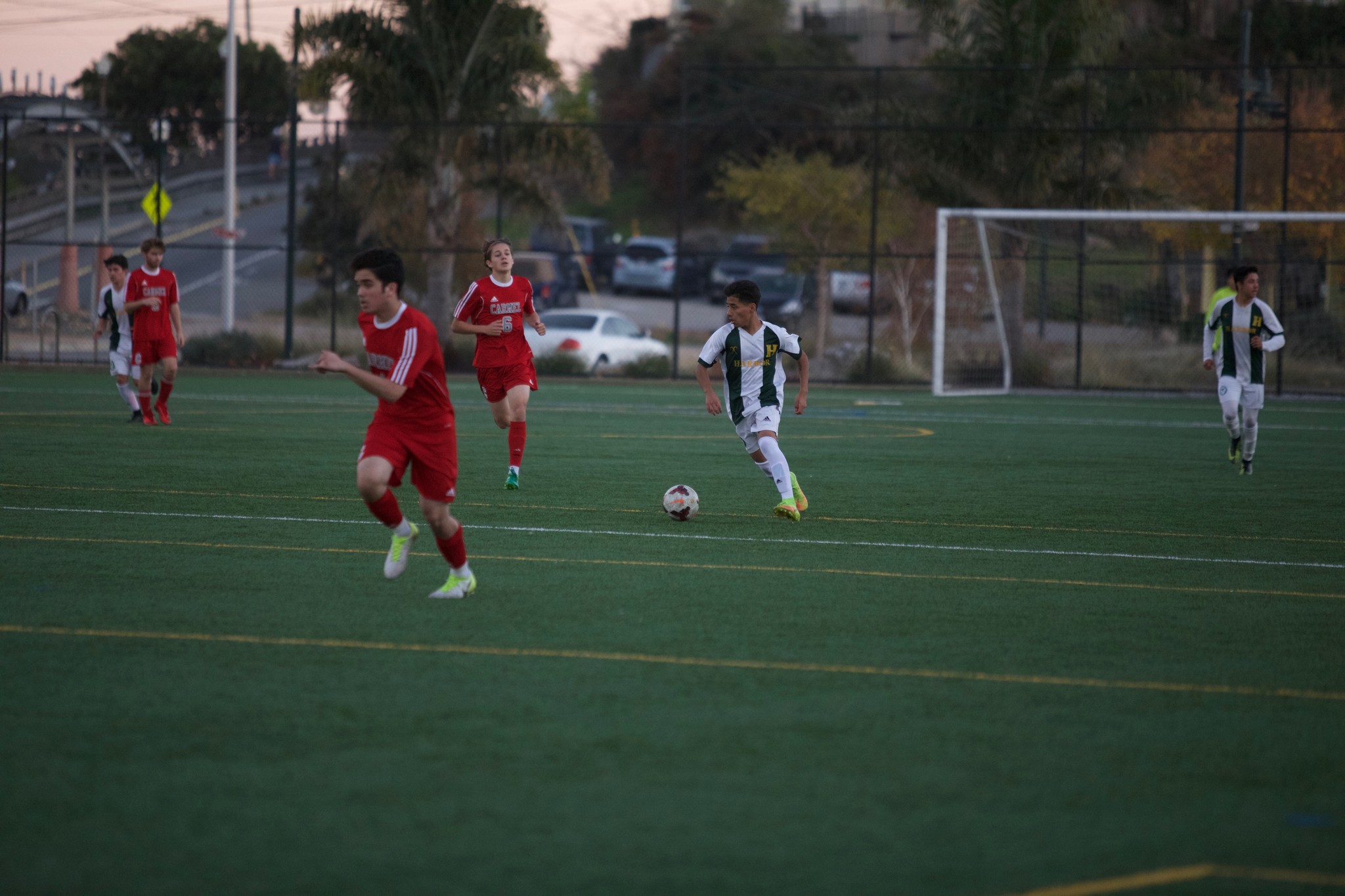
[663,485,699,521]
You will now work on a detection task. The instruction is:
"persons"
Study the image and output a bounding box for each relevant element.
[122,237,184,424]
[452,239,547,489]
[310,248,476,599]
[1204,266,1285,474]
[696,279,809,523]
[92,255,159,423]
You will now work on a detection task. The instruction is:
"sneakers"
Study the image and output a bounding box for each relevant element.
[790,471,808,512]
[1239,462,1253,476]
[773,500,800,521]
[154,398,171,424]
[143,409,157,425]
[383,522,419,579]
[1228,436,1241,463]
[504,466,519,490]
[429,571,476,599]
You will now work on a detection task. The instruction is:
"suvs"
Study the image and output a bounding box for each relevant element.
[613,235,679,296]
[513,215,614,313]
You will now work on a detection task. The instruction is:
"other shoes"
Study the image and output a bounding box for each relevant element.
[126,414,143,423]
[150,377,159,395]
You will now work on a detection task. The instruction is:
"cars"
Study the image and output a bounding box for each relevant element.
[828,266,879,314]
[518,310,672,377]
[3,278,30,317]
[709,235,813,308]
[922,265,1003,319]
